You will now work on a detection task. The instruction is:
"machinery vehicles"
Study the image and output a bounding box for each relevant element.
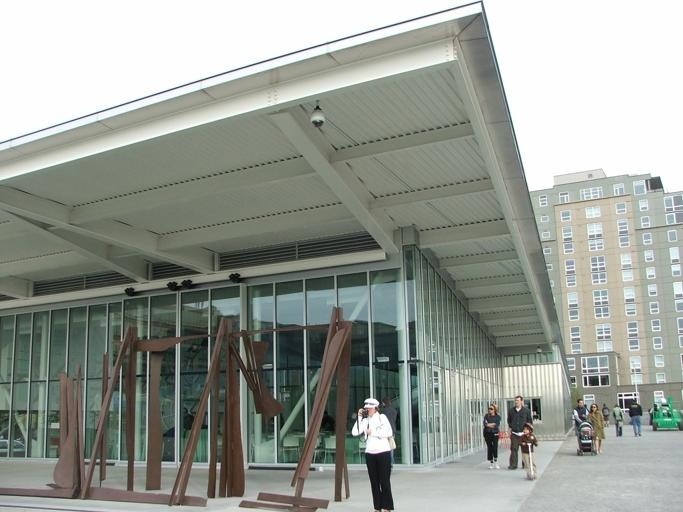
[653,397,683,430]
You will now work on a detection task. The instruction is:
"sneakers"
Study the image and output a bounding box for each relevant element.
[508,465,517,469]
[488,463,500,469]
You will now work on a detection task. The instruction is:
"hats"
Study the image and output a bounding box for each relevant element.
[363,398,379,409]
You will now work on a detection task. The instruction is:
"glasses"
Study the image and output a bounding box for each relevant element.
[488,408,493,410]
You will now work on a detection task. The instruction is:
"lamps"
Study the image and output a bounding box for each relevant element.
[282,431,367,463]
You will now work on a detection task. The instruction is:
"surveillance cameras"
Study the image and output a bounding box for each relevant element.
[310,111,326,128]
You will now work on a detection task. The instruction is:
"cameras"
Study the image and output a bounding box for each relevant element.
[362,411,369,418]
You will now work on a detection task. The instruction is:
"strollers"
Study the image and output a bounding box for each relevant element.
[577,421,599,455]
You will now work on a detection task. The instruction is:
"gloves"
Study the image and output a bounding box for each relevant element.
[366,430,371,435]
[358,409,364,416]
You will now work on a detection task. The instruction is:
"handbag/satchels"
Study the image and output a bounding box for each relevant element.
[618,422,623,427]
[388,436,396,450]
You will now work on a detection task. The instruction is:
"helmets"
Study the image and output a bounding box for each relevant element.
[525,423,533,432]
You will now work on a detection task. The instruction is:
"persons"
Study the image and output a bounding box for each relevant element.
[573,398,642,454]
[183,407,195,438]
[381,397,398,466]
[507,396,532,470]
[483,404,502,469]
[322,411,335,432]
[518,423,538,479]
[352,398,394,512]
[648,405,654,425]
